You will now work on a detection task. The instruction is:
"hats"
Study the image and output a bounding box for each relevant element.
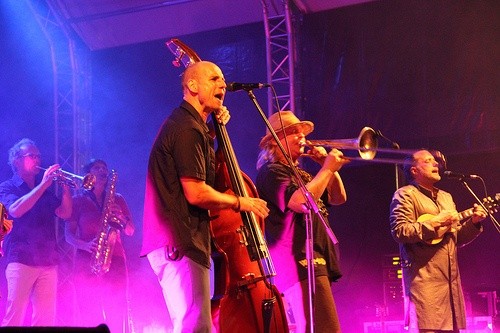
[258,111,314,149]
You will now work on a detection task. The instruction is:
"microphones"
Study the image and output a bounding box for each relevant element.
[375,129,399,150]
[444,170,479,180]
[227,80,271,92]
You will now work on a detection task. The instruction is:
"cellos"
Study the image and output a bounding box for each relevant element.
[167,37,290,333]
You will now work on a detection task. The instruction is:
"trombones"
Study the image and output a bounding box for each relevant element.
[296,126,445,168]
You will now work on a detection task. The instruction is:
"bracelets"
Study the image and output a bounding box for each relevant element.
[232,193,241,213]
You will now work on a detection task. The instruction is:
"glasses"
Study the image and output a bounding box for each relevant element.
[20,152,43,161]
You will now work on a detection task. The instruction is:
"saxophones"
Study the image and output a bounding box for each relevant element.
[89,168,124,278]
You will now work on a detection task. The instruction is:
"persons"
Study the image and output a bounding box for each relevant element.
[0,138,74,327]
[0,218,15,239]
[254,111,348,333]
[139,61,271,332]
[65,159,137,333]
[391,149,488,333]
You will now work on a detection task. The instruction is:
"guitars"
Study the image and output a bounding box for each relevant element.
[413,193,500,245]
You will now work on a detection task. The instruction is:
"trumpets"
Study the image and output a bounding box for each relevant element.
[36,164,97,192]
